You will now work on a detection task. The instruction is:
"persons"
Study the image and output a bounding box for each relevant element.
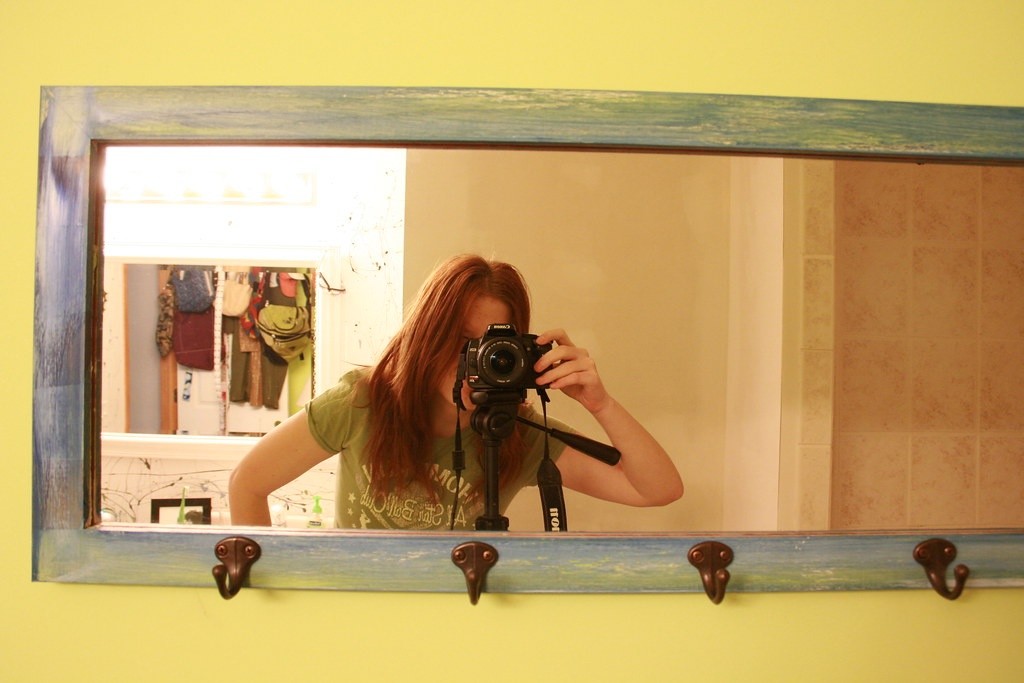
[231,252,683,532]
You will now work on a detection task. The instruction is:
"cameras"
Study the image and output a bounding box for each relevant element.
[465,322,554,388]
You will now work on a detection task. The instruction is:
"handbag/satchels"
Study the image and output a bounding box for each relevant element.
[256,302,311,360]
[212,280,254,316]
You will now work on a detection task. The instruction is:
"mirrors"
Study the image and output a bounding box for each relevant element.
[31,83,1024,592]
[123,264,316,436]
